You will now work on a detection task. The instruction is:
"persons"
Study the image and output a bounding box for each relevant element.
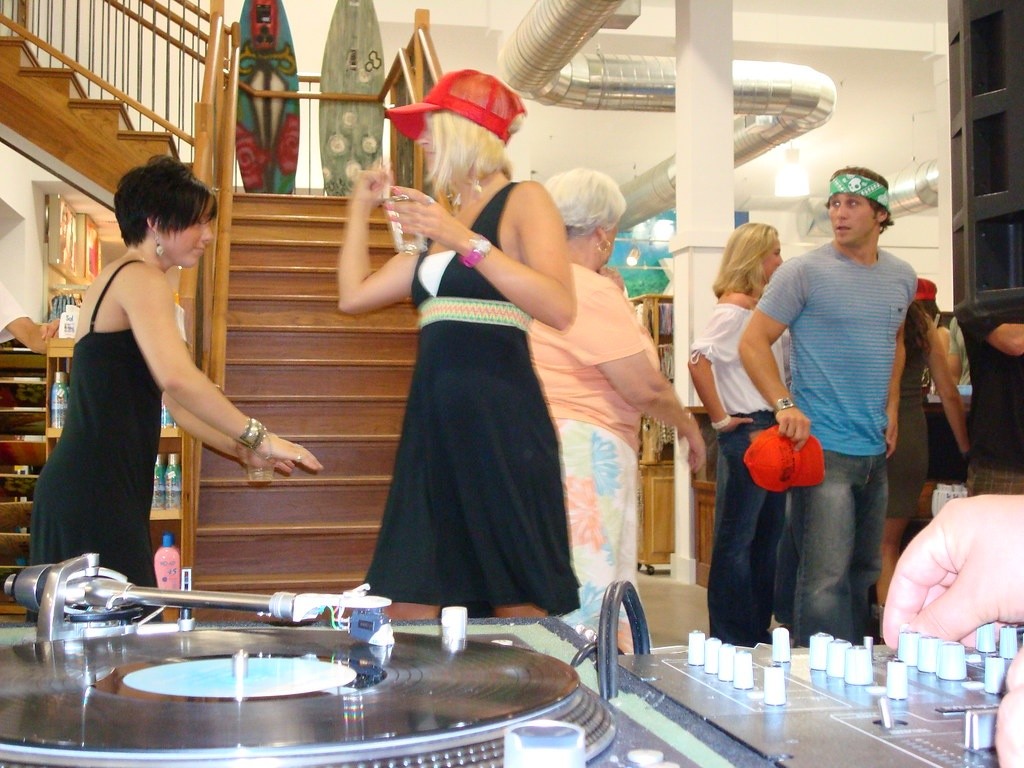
[687,223,1024,648]
[519,169,705,654]
[740,167,919,648]
[337,69,582,620]
[0,154,324,623]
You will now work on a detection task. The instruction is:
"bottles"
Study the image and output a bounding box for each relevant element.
[154,533,181,591]
[161,399,176,425]
[150,451,182,511]
[51,371,70,429]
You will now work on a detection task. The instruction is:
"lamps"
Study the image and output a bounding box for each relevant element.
[625,245,641,266]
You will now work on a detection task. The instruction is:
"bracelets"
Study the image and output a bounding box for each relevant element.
[250,434,273,461]
[962,451,970,462]
[712,415,731,430]
[240,417,267,447]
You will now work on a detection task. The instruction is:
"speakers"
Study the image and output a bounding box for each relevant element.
[949,0,1024,323]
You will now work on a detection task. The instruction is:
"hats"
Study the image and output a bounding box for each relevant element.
[743,425,825,493]
[384,69,527,142]
[913,279,937,300]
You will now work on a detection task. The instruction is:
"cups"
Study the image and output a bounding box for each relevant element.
[247,448,274,482]
[385,194,429,255]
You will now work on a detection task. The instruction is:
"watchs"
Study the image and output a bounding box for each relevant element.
[774,397,798,414]
[459,233,492,269]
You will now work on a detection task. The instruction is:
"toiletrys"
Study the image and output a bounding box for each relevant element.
[155,532,181,591]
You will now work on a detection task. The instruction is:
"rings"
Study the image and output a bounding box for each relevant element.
[296,454,302,464]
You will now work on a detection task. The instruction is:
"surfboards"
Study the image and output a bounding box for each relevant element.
[319,0,385,196]
[236,0,300,194]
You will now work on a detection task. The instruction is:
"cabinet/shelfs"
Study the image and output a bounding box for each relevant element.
[0,338,182,612]
